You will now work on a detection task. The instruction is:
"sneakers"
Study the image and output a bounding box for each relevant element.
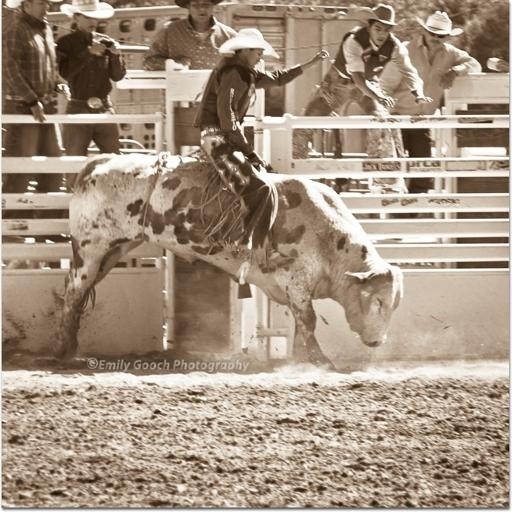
[259,247,294,273]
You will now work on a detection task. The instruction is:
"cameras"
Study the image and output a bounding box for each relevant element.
[97,36,114,49]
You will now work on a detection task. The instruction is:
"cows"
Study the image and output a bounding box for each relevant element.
[39,152,405,374]
[333,90,408,220]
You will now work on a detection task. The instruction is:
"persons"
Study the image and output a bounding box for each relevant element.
[143,0,238,70]
[379,10,482,218]
[192,28,330,273]
[2,0,71,245]
[55,0,126,193]
[293,4,434,159]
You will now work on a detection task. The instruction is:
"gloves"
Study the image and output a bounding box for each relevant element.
[247,152,263,167]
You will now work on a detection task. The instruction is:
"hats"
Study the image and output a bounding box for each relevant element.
[59,1,114,19]
[348,4,398,26]
[174,0,223,8]
[218,29,280,59]
[412,11,463,37]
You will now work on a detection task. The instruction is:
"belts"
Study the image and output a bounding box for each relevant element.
[199,128,221,137]
[173,102,197,108]
[4,94,56,104]
